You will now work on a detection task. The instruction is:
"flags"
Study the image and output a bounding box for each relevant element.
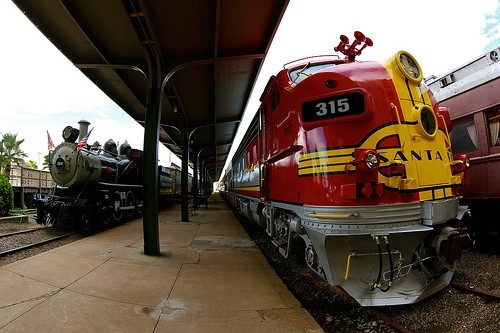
[76,128,95,151]
[47,131,55,150]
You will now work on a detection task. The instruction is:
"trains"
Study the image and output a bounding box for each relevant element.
[217,29,500,308]
[32,120,199,237]
